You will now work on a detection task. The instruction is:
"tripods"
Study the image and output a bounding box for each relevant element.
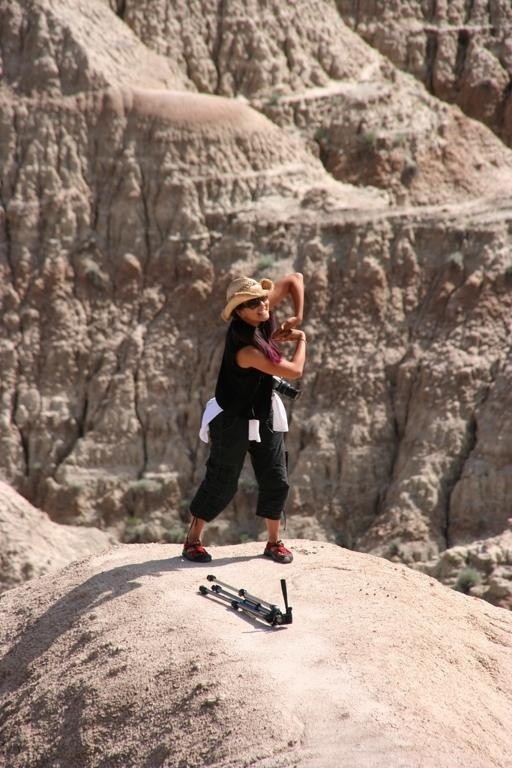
[198,574,294,627]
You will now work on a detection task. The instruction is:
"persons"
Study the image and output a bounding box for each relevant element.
[182,272,307,563]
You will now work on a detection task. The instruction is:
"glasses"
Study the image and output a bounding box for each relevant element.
[238,298,266,310]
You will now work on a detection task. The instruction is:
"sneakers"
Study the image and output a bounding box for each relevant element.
[264,540,293,563]
[182,538,211,562]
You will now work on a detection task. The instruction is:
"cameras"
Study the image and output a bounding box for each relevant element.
[271,375,301,400]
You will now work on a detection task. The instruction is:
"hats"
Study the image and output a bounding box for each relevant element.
[220,277,274,323]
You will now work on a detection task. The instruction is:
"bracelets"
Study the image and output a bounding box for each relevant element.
[297,338,308,345]
